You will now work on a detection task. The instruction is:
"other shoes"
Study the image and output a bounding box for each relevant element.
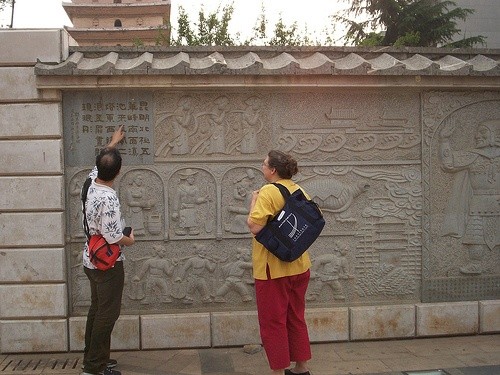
[285,368,311,375]
[82,367,121,375]
[81,359,117,369]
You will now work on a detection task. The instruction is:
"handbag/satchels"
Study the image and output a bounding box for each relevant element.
[89,234,122,270]
[254,182,327,263]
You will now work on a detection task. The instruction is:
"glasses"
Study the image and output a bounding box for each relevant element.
[261,159,271,170]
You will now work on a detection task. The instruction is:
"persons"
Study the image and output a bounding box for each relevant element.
[70,95,500,305]
[246,149,322,375]
[81,124,134,375]
